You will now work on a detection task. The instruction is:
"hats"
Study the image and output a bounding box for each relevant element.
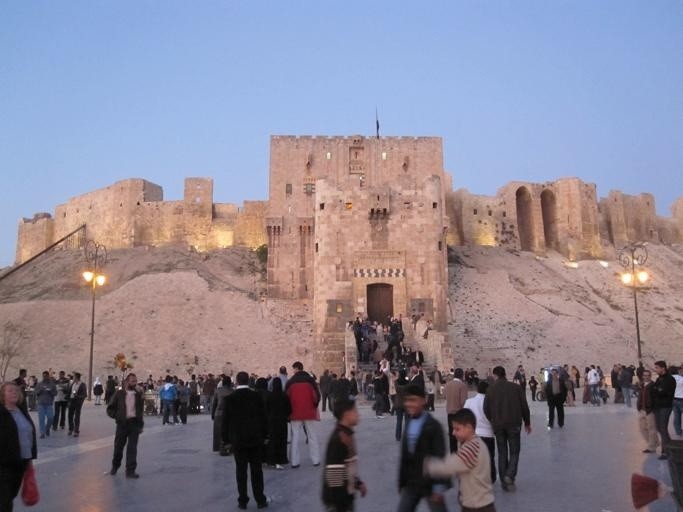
[399,384,425,396]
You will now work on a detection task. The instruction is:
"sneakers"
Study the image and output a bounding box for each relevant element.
[103,468,116,475]
[40,425,80,438]
[165,419,186,425]
[257,496,271,507]
[263,457,300,469]
[126,470,140,478]
[658,455,668,460]
[504,476,515,492]
[313,462,321,466]
[642,449,656,452]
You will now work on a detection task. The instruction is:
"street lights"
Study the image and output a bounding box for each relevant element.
[618,243,652,372]
[81,239,107,401]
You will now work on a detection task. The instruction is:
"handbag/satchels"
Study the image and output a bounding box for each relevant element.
[23,461,39,505]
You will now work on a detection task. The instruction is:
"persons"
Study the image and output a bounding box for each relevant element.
[349,314,424,368]
[611,360,683,459]
[319,359,531,511]
[528,364,606,428]
[93,373,216,478]
[0,367,88,511]
[212,362,320,510]
